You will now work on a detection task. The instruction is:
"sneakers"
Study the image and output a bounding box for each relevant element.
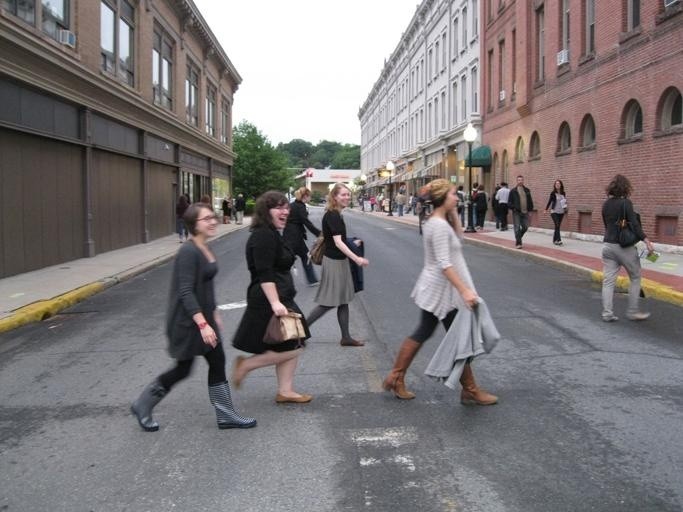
[517,245,522,249]
[553,240,562,245]
[626,313,649,320]
[603,316,618,321]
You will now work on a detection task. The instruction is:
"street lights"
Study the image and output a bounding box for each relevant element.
[361,174,367,211]
[349,180,355,210]
[386,162,395,217]
[461,124,480,233]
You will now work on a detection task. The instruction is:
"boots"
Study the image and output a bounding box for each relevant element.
[383,337,423,399]
[208,381,256,429]
[460,366,497,404]
[131,380,167,431]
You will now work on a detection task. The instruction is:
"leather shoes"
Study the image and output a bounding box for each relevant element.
[341,339,364,345]
[232,357,242,387]
[276,392,311,403]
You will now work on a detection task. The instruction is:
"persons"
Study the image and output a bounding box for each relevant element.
[355,174,569,250]
[176,189,247,245]
[599,172,656,322]
[379,177,501,407]
[231,190,313,404]
[304,182,370,347]
[129,202,258,432]
[283,186,322,288]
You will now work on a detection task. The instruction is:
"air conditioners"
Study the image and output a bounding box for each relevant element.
[59,29,75,48]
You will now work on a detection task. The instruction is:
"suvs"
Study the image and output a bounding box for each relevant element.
[310,194,329,204]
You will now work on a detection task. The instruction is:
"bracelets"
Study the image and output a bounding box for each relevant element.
[197,321,209,329]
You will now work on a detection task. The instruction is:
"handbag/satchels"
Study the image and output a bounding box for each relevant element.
[616,214,642,248]
[310,234,325,265]
[262,309,293,344]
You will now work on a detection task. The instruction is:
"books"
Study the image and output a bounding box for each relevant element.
[639,247,660,263]
